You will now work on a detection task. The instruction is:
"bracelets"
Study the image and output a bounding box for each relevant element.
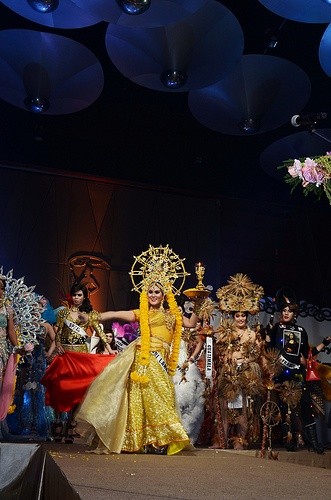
[89,310,102,321]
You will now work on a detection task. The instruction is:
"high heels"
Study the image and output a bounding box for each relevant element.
[54,423,63,442]
[64,423,75,444]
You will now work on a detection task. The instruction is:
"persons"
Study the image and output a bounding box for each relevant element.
[265,303,324,454]
[208,309,261,451]
[42,284,120,444]
[173,304,207,447]
[76,281,204,455]
[7,298,57,441]
[309,336,331,359]
[96,328,118,354]
[0,274,25,439]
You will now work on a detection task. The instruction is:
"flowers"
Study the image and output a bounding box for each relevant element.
[277,152,331,207]
